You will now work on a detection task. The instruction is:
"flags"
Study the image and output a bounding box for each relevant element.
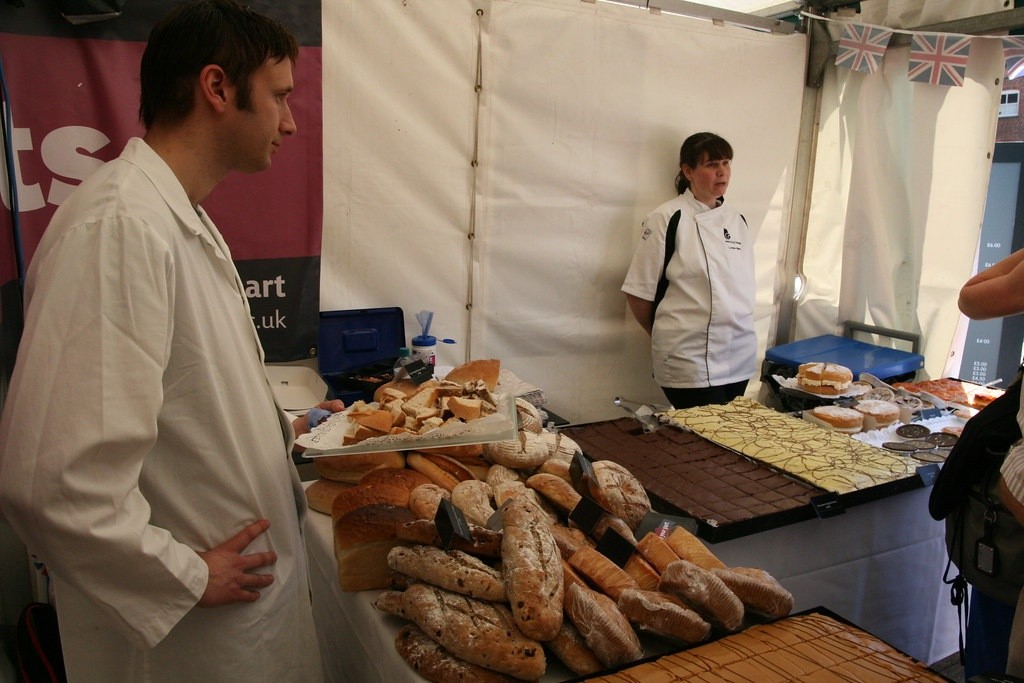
[833,25,891,75]
[1001,35,1024,78]
[907,35,972,87]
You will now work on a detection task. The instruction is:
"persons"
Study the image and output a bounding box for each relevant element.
[0,0,322,683]
[958,248,1024,683]
[621,132,757,410]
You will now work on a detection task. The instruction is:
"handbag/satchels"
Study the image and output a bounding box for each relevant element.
[945,478,1024,606]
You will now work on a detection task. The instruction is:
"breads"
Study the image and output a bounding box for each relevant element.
[300,358,794,683]
[892,378,1007,410]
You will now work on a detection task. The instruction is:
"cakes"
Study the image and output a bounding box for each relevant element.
[811,405,863,429]
[798,362,853,396]
[852,400,900,424]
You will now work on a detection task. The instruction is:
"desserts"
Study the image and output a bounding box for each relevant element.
[882,409,981,470]
[852,382,936,414]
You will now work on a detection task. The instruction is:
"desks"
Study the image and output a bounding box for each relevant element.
[699,476,974,669]
[300,478,426,683]
